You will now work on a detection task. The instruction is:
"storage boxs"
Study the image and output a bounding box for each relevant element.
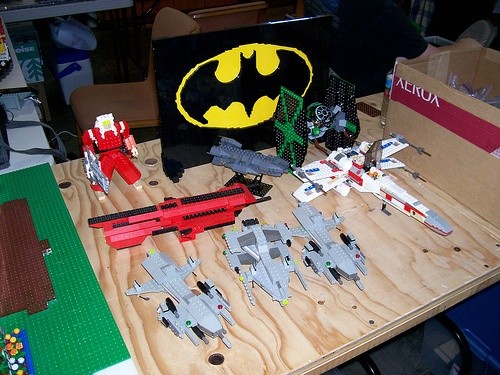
[8,25,45,84]
[434,281,500,375]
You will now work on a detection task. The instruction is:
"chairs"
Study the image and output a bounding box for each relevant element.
[426,20,498,46]
[69,7,201,153]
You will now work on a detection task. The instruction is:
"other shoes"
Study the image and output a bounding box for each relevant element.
[455,20,495,48]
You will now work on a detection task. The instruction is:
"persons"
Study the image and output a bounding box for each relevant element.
[305,0,437,93]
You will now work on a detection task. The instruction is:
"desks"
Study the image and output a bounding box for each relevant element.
[0,17,56,175]
[382,38,500,228]
[0,91,500,375]
